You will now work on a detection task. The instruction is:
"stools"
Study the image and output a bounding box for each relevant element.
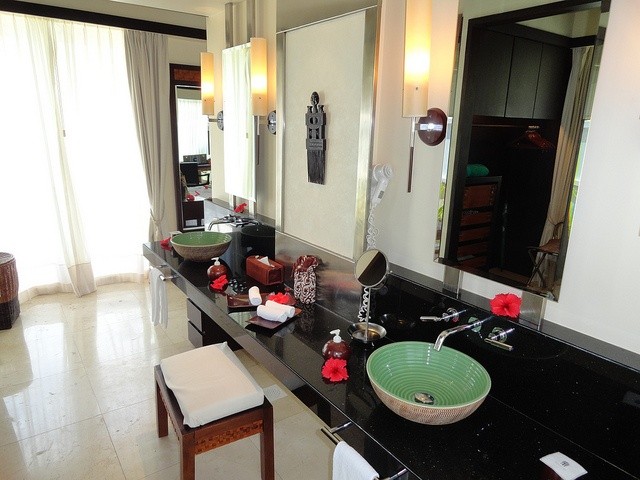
[153,342,274,480]
[1,252,20,327]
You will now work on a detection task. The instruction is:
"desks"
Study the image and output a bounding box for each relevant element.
[143,221,639,480]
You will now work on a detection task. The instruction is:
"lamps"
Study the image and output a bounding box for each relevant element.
[403,0,445,194]
[250,37,277,165]
[201,50,226,157]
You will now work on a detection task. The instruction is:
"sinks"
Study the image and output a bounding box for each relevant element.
[366,341,492,425]
[170,231,232,260]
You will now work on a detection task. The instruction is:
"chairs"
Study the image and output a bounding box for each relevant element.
[183,162,209,188]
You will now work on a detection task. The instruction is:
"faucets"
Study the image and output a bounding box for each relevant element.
[207,214,235,232]
[433,315,491,354]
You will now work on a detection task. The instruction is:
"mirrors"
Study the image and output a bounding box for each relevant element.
[439,0,605,296]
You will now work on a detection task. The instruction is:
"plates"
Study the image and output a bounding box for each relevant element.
[226,293,295,310]
[245,305,301,330]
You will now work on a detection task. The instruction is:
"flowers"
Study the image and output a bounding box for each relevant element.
[491,291,523,318]
[159,232,175,256]
[268,289,292,307]
[231,201,248,215]
[206,272,229,293]
[320,356,351,387]
